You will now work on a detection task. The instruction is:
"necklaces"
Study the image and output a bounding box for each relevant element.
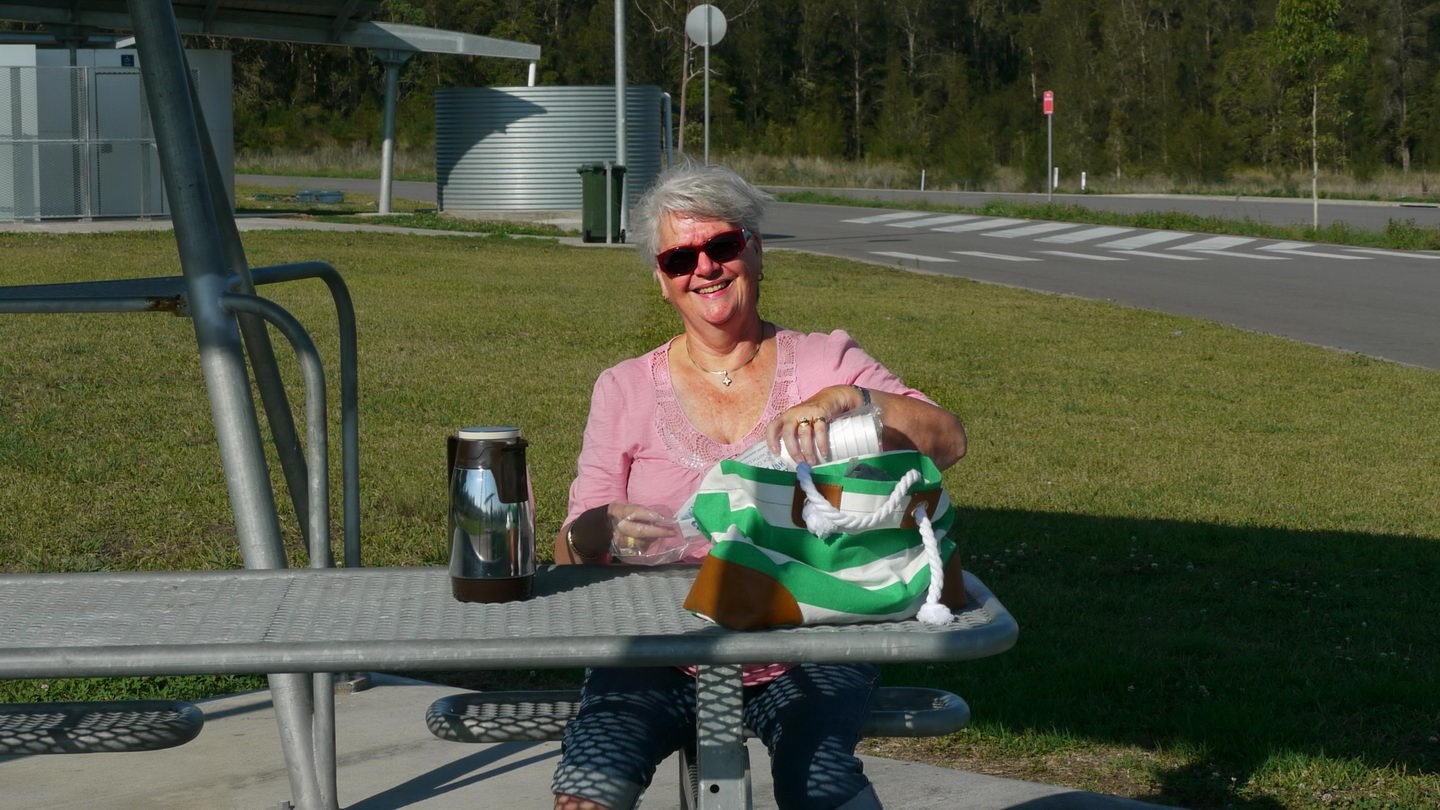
[686,323,765,386]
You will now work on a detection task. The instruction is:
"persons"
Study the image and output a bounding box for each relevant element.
[551,158,967,809]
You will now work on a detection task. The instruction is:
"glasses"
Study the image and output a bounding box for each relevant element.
[656,226,750,277]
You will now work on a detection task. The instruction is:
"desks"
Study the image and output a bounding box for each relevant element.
[1,561,1019,809]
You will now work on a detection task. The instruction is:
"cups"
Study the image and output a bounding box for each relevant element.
[780,411,880,468]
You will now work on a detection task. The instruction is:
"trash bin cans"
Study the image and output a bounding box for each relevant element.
[577,160,627,243]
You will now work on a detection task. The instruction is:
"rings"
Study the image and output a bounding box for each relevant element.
[799,420,810,425]
[814,417,825,423]
[627,537,635,548]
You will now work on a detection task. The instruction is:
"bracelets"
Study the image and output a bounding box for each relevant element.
[567,520,601,560]
[860,387,871,405]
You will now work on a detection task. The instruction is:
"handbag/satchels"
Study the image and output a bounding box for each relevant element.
[682,451,969,629]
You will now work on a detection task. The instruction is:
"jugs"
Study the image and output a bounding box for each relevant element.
[446,425,536,602]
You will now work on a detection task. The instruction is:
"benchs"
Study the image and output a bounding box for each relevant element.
[1,700,204,755]
[424,679,973,743]
